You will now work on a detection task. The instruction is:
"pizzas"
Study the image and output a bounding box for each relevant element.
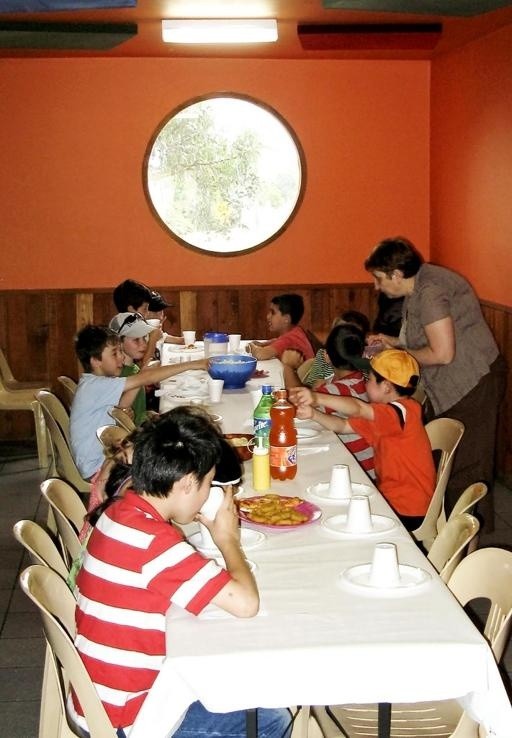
[234,495,304,513]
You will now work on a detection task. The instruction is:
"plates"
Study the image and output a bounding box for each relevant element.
[320,513,401,538]
[293,426,322,440]
[208,413,224,422]
[209,556,257,578]
[343,562,433,596]
[233,494,324,530]
[306,481,378,503]
[185,527,269,555]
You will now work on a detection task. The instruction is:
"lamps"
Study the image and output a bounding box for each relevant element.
[160,16,279,45]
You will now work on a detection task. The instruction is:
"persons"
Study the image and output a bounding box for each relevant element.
[361,238,505,536]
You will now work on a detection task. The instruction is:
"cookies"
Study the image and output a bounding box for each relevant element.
[249,504,307,525]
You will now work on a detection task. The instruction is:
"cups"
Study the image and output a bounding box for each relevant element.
[167,331,244,404]
[147,360,160,367]
[344,495,374,535]
[368,541,405,589]
[326,463,354,499]
[199,487,224,545]
[146,318,162,330]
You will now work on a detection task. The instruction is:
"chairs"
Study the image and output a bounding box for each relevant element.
[0,345,510,736]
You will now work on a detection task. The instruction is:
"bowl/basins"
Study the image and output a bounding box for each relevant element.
[220,433,254,462]
[206,354,258,389]
[364,342,382,358]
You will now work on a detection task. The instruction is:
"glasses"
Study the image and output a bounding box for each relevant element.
[118,312,146,334]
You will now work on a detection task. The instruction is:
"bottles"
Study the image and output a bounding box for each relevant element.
[268,390,300,481]
[253,384,274,452]
[247,433,272,494]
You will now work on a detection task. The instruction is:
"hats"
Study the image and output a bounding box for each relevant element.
[148,290,176,312]
[109,311,155,338]
[367,346,421,388]
